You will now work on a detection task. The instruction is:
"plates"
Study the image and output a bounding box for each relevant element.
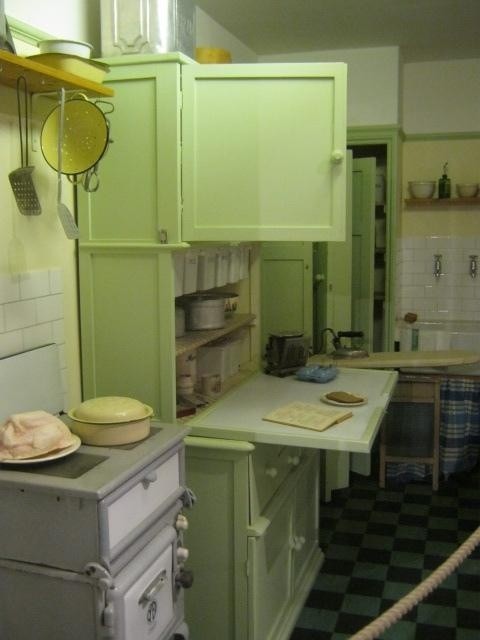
[0,431,81,466]
[320,393,368,406]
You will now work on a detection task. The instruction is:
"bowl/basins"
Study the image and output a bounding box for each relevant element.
[217,291,239,316]
[180,294,226,333]
[407,180,436,200]
[65,396,155,447]
[455,182,479,199]
[37,39,93,58]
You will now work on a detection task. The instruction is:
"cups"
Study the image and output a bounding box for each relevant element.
[201,373,222,396]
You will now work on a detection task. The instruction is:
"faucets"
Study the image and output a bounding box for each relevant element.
[433,254,441,277]
[470,255,478,278]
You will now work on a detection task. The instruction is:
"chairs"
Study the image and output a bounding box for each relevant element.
[374,378,446,494]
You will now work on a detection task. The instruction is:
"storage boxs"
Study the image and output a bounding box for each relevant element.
[195,337,244,385]
[172,246,251,298]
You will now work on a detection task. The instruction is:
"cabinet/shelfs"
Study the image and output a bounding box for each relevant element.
[183,434,325,639]
[74,48,348,418]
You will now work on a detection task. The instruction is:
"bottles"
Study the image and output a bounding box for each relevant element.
[438,174,452,199]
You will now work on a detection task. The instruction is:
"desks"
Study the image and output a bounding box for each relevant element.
[305,344,479,375]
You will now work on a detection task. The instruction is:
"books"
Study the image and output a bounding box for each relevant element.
[261,399,354,432]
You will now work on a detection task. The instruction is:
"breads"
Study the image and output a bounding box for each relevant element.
[327,391,364,403]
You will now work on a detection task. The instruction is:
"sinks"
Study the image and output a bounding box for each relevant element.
[397,320,479,352]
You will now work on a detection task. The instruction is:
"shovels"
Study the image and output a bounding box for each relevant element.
[57,87,81,240]
[8,76,42,215]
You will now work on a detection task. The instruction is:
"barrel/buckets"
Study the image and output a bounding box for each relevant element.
[197,46,232,64]
